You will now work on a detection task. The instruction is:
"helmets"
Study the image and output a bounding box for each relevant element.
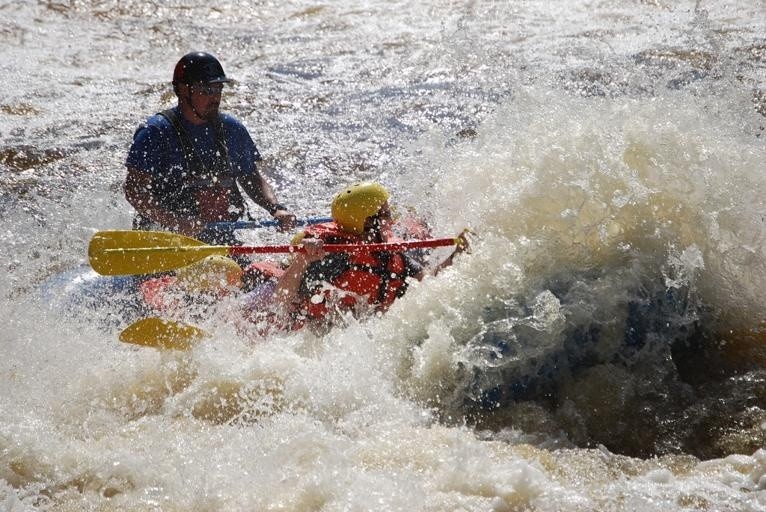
[176,255,241,295]
[172,52,230,95]
[332,183,389,235]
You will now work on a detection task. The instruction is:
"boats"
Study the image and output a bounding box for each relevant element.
[27,237,707,409]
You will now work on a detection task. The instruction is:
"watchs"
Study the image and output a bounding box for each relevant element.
[269,203,287,217]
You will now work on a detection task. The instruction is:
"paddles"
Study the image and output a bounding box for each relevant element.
[119,317,207,349]
[89,229,471,275]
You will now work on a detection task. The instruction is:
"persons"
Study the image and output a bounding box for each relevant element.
[168,203,436,332]
[124,50,298,291]
[272,183,477,335]
